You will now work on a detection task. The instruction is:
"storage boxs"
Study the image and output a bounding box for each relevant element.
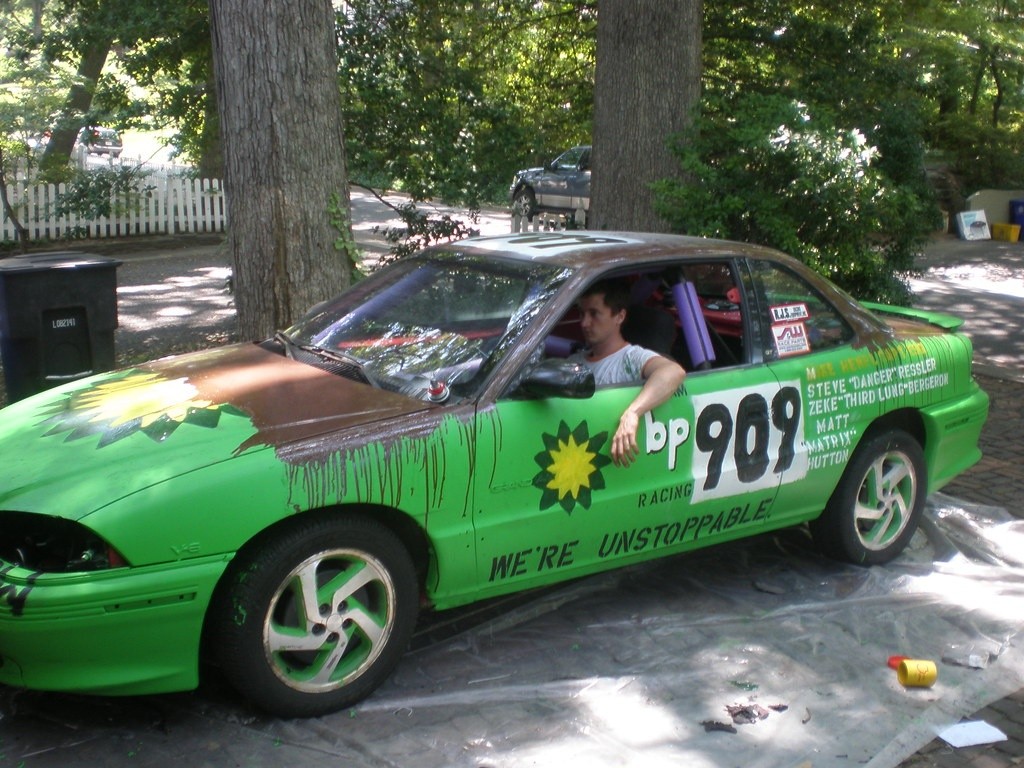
[993,221,1021,243]
[955,208,992,241]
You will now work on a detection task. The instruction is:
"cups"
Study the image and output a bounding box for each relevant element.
[898,659,937,688]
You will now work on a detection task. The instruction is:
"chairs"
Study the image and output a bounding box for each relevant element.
[622,305,675,355]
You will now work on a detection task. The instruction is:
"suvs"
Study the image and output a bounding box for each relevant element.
[505,144,595,223]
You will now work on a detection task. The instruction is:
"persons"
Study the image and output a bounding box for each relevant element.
[565,281,687,468]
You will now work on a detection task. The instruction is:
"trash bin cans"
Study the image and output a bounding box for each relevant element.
[0,251,124,405]
[1009,199,1024,241]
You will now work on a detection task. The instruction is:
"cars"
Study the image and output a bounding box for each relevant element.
[77,126,123,159]
[0,224,990,720]
